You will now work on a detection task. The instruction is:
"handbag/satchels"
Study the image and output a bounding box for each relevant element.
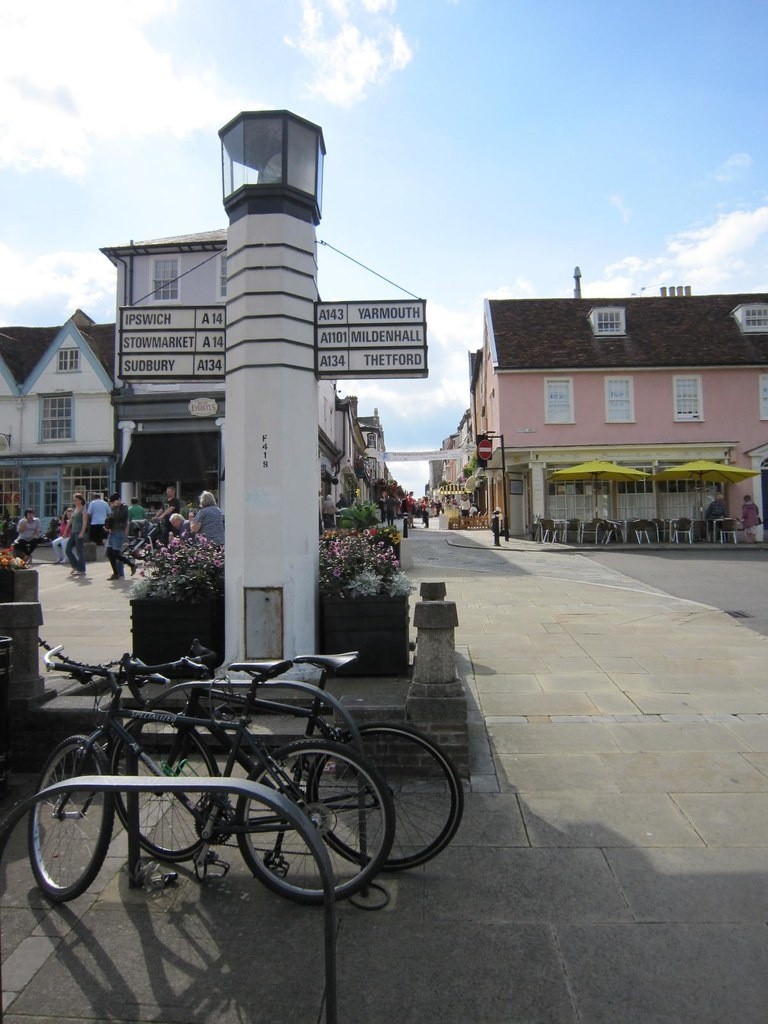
[425,508,431,512]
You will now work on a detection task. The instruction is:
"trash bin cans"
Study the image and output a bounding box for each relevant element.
[0,635,14,798]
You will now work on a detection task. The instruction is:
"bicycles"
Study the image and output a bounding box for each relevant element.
[104,637,465,875]
[26,644,394,906]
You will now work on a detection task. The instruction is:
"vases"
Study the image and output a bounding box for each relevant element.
[129,585,225,678]
[321,592,413,680]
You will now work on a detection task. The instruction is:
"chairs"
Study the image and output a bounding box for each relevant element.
[536,517,738,545]
[473,510,478,516]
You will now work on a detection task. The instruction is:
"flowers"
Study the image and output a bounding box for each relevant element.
[320,526,412,599]
[0,547,32,571]
[130,531,226,604]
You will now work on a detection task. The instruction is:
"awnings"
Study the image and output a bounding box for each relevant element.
[116,431,220,491]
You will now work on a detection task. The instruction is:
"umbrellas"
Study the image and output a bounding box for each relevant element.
[648,460,762,520]
[545,460,651,519]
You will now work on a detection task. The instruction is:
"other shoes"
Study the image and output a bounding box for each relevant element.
[119,573,123,576]
[412,526,416,528]
[55,559,62,563]
[107,574,119,581]
[60,559,69,563]
[130,565,138,576]
[71,569,84,574]
[26,556,32,564]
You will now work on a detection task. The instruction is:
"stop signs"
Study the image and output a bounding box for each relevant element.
[478,440,493,460]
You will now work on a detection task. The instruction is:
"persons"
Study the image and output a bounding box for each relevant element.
[51,508,75,563]
[469,503,479,517]
[167,512,197,540]
[125,497,146,522]
[12,509,42,565]
[322,494,336,529]
[103,493,138,580]
[375,491,457,528]
[124,530,143,554]
[741,495,760,543]
[460,492,470,525]
[151,485,181,546]
[62,493,88,576]
[335,493,351,508]
[188,489,225,551]
[86,495,112,546]
[704,494,727,543]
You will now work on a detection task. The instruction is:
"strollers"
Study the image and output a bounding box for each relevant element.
[121,519,160,564]
